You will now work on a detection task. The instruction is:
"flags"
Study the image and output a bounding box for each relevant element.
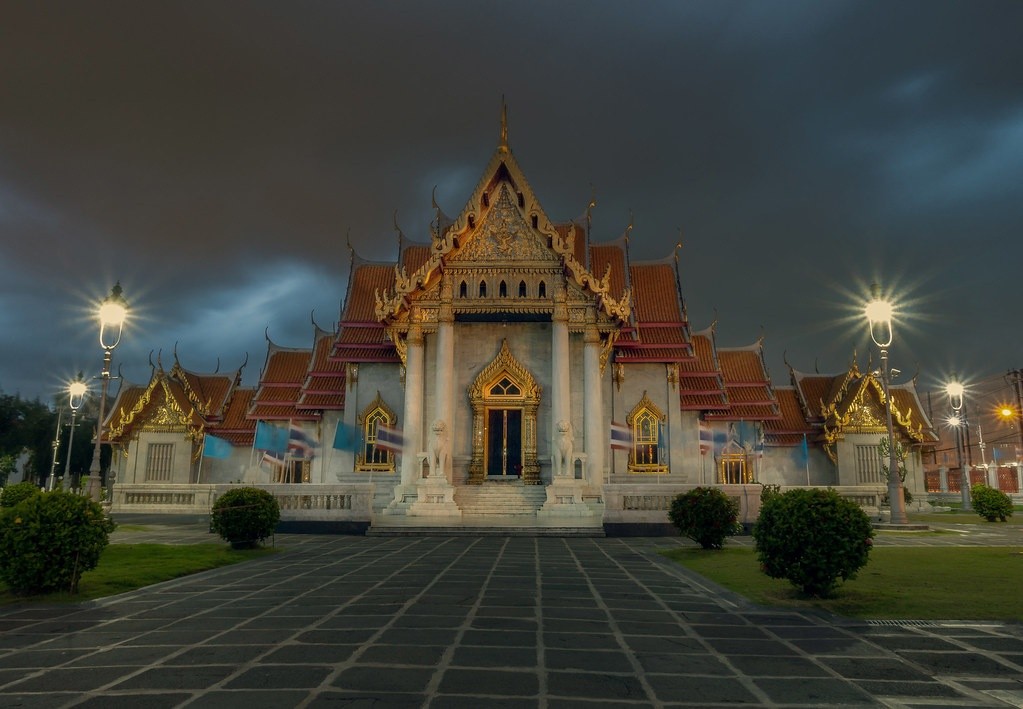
[202,418,404,468]
[1015,445,1023,458]
[607,417,809,471]
[993,447,1004,459]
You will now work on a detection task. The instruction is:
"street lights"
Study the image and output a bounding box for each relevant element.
[62,369,86,494]
[865,285,907,522]
[84,278,129,503]
[947,371,970,510]
